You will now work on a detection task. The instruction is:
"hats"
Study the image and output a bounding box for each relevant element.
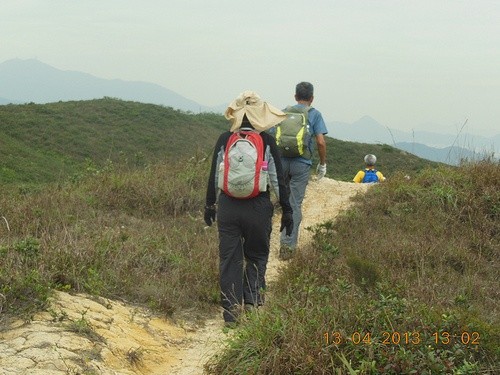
[224,90,287,134]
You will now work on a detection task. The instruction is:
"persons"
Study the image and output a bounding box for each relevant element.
[203,92,294,333]
[271,82,328,259]
[353,154,384,184]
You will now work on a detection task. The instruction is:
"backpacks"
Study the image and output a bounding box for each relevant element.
[275,105,316,158]
[361,168,379,184]
[217,125,269,199]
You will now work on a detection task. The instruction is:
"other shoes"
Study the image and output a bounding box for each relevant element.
[222,321,240,333]
[280,246,299,261]
[245,302,256,312]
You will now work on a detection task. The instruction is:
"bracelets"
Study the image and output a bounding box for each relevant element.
[205,203,217,210]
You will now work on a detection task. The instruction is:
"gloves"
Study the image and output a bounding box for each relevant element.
[205,204,215,226]
[315,160,327,181]
[278,209,294,236]
